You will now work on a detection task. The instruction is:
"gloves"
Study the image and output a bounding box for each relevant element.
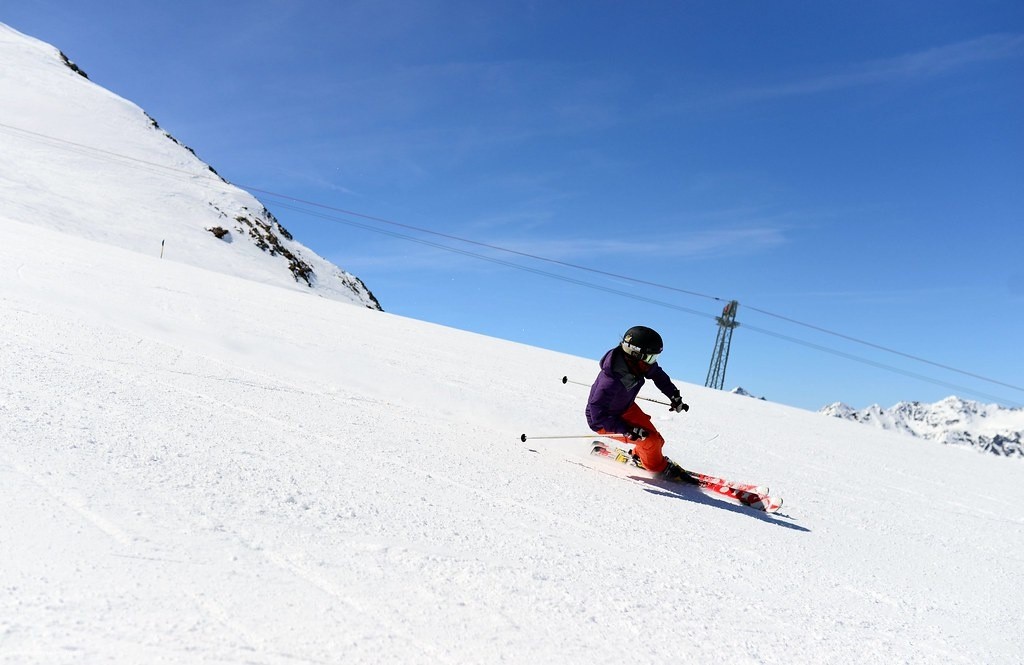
[670,389,683,413]
[628,426,646,442]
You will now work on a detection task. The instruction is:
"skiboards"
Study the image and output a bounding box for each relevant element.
[590,440,784,515]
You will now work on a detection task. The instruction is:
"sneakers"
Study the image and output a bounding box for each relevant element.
[652,455,699,485]
[628,449,642,466]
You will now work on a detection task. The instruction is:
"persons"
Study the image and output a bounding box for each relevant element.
[585,325,686,476]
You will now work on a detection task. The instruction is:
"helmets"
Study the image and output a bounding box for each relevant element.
[620,326,663,366]
